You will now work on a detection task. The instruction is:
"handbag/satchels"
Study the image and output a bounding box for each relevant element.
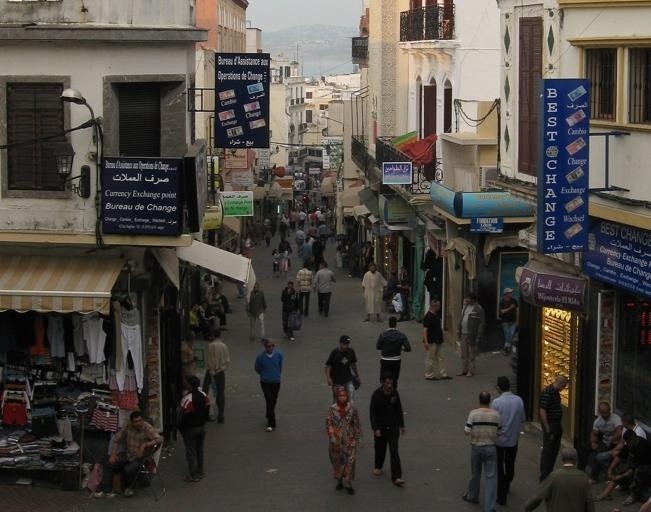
[294,312,301,330]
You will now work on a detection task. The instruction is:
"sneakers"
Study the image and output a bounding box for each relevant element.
[344,486,354,495]
[184,472,207,482]
[267,426,273,432]
[463,491,479,504]
[425,369,472,381]
[373,467,382,475]
[95,492,114,499]
[336,479,343,490]
[124,488,134,496]
[393,478,405,485]
[590,478,636,505]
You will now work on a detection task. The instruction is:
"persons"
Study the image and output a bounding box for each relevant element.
[237,282,267,342]
[593,430,650,501]
[348,240,411,323]
[369,370,407,485]
[244,220,272,261]
[638,498,651,511]
[325,334,358,403]
[375,315,413,381]
[421,300,453,381]
[279,207,332,257]
[488,376,527,505]
[326,386,362,494]
[272,235,337,341]
[181,270,231,423]
[176,374,211,483]
[253,335,283,433]
[500,287,518,355]
[80,400,162,499]
[591,401,623,459]
[455,292,484,378]
[539,374,569,480]
[522,447,596,511]
[459,390,502,511]
[292,169,323,212]
[594,413,647,479]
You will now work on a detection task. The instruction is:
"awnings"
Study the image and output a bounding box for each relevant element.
[353,205,371,224]
[176,238,257,303]
[222,216,240,235]
